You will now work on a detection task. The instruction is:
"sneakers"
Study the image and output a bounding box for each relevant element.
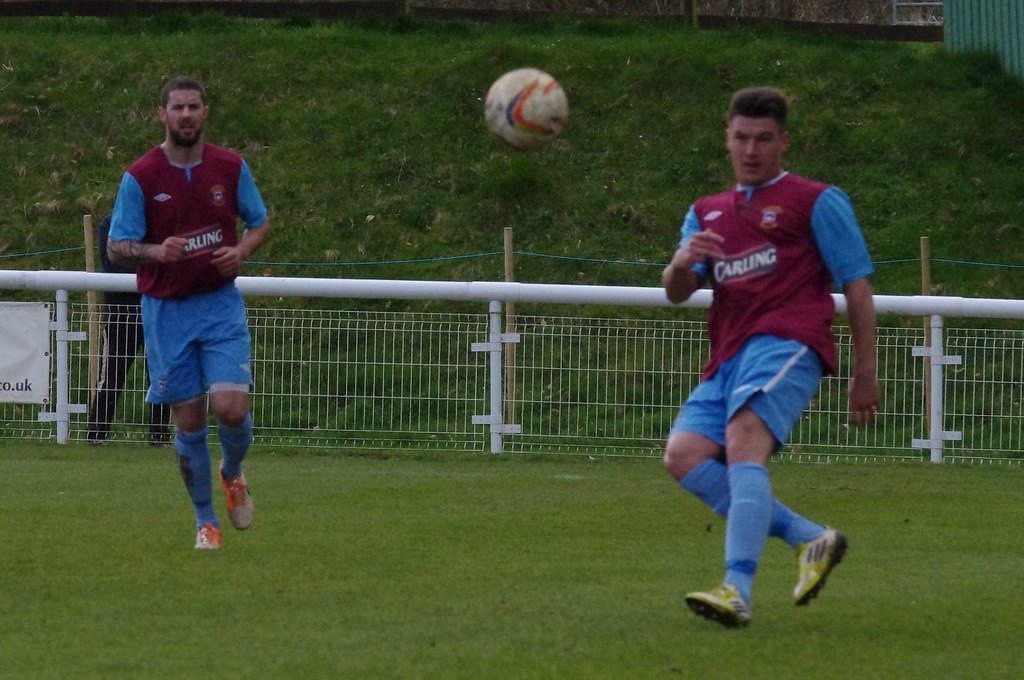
[218,460,253,530]
[794,524,846,605]
[686,581,751,628]
[195,522,223,550]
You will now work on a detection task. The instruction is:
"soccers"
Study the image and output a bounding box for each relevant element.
[484,66,570,151]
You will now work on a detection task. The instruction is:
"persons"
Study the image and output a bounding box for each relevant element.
[86,213,171,447]
[660,86,881,631]
[106,76,271,551]
